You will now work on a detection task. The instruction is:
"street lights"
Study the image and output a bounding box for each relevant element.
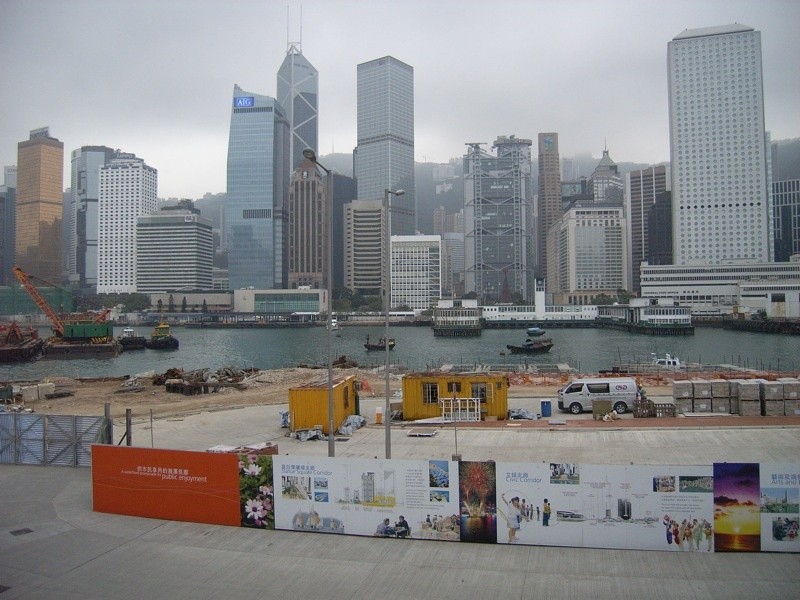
[301,145,336,459]
[383,188,406,459]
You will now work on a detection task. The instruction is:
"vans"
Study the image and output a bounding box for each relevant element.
[556,375,639,414]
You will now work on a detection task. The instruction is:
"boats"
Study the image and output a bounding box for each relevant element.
[144,313,180,350]
[363,333,396,352]
[526,327,546,337]
[506,337,553,356]
[115,324,146,350]
[325,319,339,331]
[649,352,680,370]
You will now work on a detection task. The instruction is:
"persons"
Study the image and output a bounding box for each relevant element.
[773,517,799,541]
[664,518,713,553]
[395,515,410,538]
[502,497,551,544]
[376,518,394,537]
[638,384,647,403]
[426,513,460,532]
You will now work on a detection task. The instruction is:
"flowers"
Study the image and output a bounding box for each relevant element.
[239,455,275,530]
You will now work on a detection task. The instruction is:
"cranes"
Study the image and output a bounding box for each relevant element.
[11,263,112,344]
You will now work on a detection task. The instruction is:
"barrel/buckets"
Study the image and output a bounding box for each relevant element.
[541,401,551,417]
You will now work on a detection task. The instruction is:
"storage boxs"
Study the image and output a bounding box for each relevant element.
[313,425,324,433]
[485,416,497,421]
[632,378,800,418]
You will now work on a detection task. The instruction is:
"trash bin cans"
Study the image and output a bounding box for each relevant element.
[541,400,551,417]
[592,399,612,418]
